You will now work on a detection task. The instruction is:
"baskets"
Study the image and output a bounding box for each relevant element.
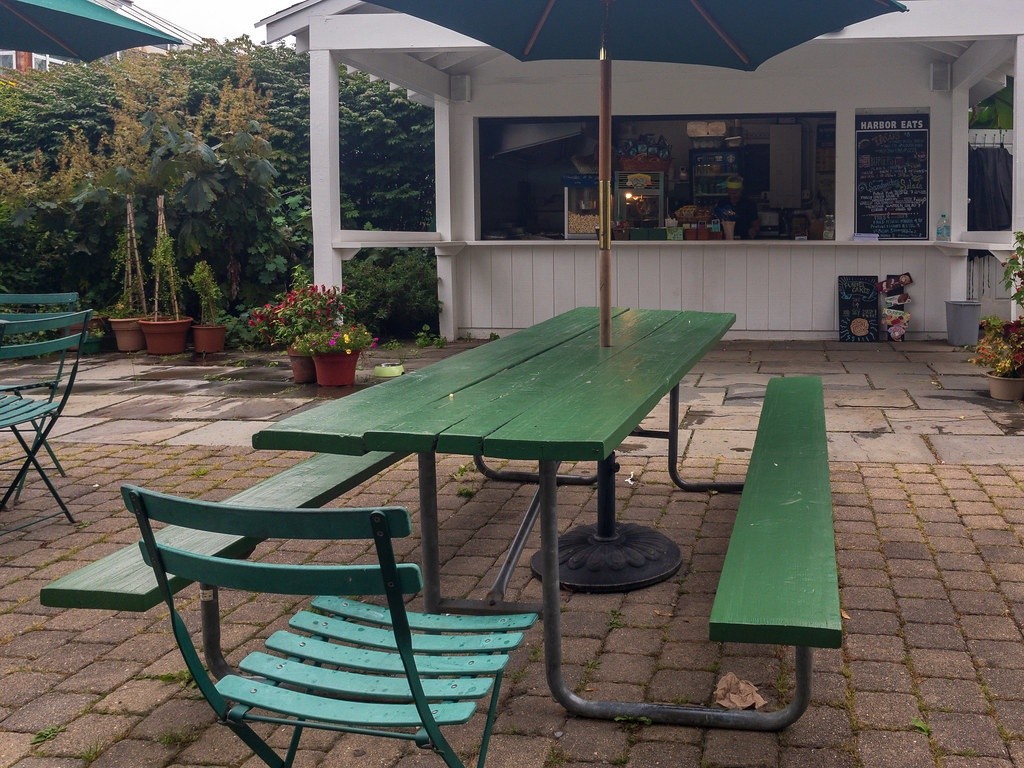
[675,202,714,223]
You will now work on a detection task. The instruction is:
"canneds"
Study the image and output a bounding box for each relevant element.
[696,164,728,194]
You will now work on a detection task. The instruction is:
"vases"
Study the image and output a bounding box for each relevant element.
[312,351,360,387]
[987,372,1024,400]
[288,351,317,383]
[61,316,115,354]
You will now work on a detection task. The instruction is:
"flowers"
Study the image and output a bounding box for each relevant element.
[249,264,379,355]
[968,314,1024,377]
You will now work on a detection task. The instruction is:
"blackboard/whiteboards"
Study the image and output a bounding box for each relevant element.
[855,114,929,240]
[838,275,880,343]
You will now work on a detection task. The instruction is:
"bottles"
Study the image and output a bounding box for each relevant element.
[936,215,951,242]
[823,215,836,241]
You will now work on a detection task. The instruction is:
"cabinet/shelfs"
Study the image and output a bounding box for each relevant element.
[692,165,738,203]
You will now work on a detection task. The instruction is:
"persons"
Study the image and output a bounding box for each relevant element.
[716,177,758,236]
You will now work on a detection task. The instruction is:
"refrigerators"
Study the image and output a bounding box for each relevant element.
[690,148,739,218]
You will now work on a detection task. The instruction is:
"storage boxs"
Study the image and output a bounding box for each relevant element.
[612,228,723,240]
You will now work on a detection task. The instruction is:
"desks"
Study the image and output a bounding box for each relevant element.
[252,306,737,726]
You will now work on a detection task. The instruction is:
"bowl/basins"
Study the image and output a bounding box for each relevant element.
[373,362,405,377]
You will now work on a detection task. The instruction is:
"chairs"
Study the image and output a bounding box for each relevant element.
[121,484,538,768]
[0,292,93,505]
[1,308,92,523]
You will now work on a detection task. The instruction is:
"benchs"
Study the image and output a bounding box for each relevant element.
[40,451,413,681]
[710,376,844,731]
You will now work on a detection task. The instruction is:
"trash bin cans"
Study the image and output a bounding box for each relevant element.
[945,301,981,347]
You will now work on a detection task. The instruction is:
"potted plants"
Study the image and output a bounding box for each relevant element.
[106,225,227,354]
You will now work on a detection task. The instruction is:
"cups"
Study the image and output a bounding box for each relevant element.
[801,189,810,199]
[721,221,735,241]
[683,229,697,240]
[789,211,808,241]
[697,227,712,241]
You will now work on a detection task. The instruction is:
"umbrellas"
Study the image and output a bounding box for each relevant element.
[0,0,184,64]
[364,0,909,346]
